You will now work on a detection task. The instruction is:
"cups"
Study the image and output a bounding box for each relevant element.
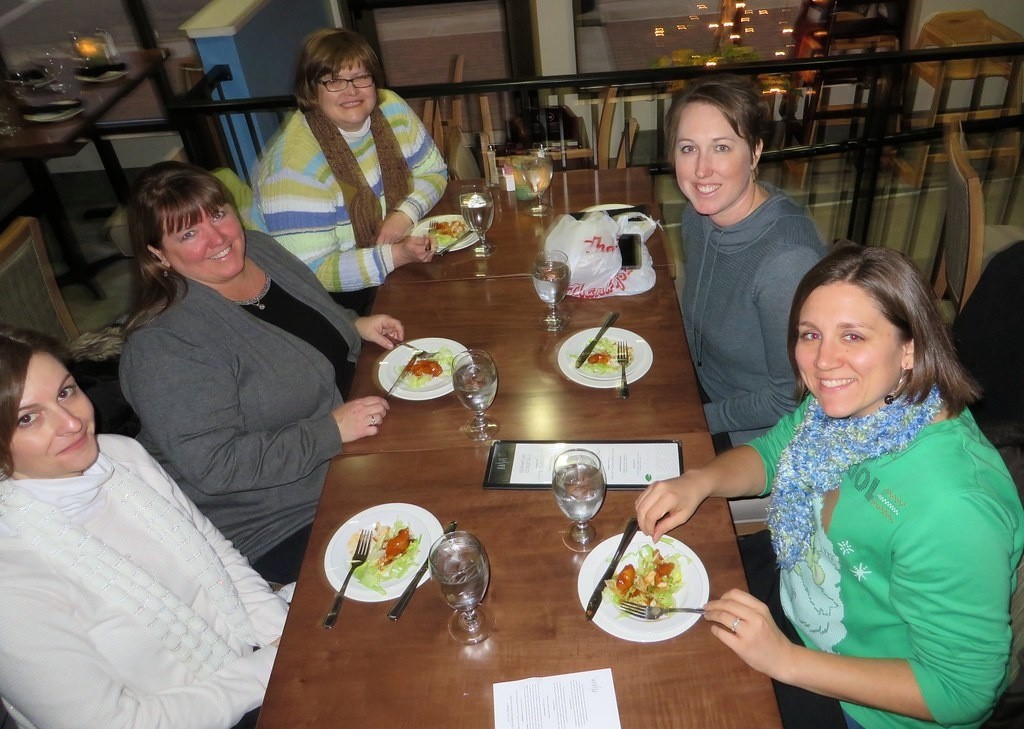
[32,47,71,92]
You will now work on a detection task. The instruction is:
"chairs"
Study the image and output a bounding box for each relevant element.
[418,56,640,180]
[930,120,1023,315]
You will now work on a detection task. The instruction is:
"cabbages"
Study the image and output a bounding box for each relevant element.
[429,230,460,245]
[399,348,458,389]
[350,520,420,596]
[567,336,622,373]
[593,537,685,620]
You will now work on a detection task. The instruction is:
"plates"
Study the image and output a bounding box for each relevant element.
[557,326,653,389]
[23,101,84,122]
[411,214,479,251]
[578,531,710,643]
[378,338,472,401]
[578,204,636,212]
[76,72,126,81]
[324,502,445,602]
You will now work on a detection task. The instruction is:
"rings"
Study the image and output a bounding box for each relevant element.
[732,617,741,632]
[371,415,376,426]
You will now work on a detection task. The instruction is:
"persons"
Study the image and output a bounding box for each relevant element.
[635,247,1024,729]
[120,159,405,586]
[0,325,298,729]
[250,26,448,318]
[665,75,831,457]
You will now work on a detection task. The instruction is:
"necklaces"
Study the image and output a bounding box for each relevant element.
[223,261,265,310]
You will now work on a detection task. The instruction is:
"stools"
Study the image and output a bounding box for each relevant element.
[775,0,1024,191]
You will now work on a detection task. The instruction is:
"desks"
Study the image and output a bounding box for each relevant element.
[0,47,172,301]
[251,166,782,728]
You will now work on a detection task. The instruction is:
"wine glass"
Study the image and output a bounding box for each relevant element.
[522,155,553,217]
[452,349,500,441]
[457,185,496,256]
[428,531,493,643]
[532,250,571,331]
[553,449,605,553]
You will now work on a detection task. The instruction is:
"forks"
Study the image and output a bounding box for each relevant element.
[322,530,373,630]
[617,340,630,399]
[382,332,438,358]
[619,599,705,620]
[425,221,435,252]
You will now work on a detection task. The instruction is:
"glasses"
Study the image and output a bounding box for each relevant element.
[318,74,373,92]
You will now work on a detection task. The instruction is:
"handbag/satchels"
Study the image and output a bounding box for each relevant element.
[544,210,656,298]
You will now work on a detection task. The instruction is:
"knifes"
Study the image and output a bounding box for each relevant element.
[575,312,620,369]
[387,520,458,621]
[436,230,473,256]
[584,518,639,624]
[383,354,417,401]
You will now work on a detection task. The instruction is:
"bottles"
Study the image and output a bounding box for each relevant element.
[486,145,499,185]
[551,142,581,171]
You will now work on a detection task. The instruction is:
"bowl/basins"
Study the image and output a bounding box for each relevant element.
[496,157,554,200]
